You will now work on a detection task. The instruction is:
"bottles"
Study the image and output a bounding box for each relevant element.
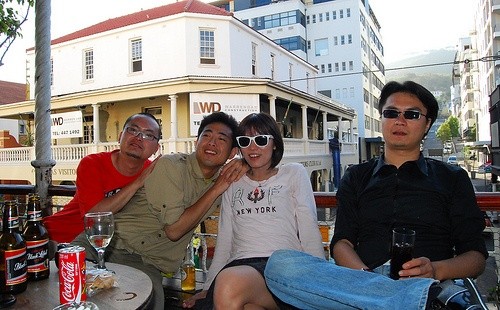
[23,192,49,282]
[181,243,196,291]
[0,199,27,293]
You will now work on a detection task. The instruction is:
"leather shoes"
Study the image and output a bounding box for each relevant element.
[430,277,488,310]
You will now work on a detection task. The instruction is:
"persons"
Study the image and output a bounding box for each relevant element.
[44,112,163,244]
[183,112,327,310]
[264,80,490,310]
[53,112,251,290]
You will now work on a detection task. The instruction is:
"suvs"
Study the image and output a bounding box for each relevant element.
[443,143,452,154]
[448,156,458,167]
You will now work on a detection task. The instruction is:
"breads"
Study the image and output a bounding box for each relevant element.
[86,269,116,289]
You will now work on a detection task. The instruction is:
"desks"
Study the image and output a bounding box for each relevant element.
[0,260,153,310]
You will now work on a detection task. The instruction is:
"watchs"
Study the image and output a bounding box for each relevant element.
[361,267,374,272]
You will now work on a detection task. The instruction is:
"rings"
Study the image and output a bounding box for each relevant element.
[235,170,240,173]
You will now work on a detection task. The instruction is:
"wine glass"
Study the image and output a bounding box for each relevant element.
[84,212,115,274]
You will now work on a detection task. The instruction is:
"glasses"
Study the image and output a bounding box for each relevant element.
[124,126,159,141]
[381,110,427,121]
[236,135,275,149]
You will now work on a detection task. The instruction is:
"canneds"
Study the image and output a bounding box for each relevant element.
[59,245,87,304]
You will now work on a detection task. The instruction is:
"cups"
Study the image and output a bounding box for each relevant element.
[391,227,416,274]
